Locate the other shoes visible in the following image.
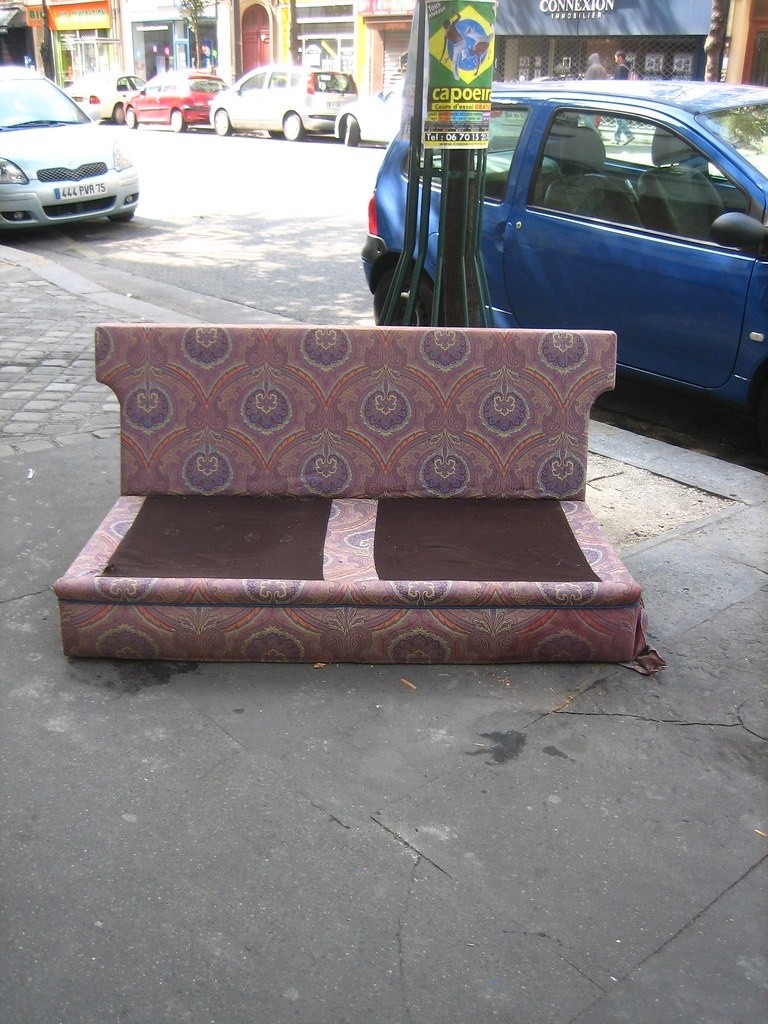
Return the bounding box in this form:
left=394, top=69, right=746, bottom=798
left=624, top=136, right=635, bottom=146
left=609, top=139, right=620, bottom=145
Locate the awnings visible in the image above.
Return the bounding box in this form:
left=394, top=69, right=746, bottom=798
left=0, top=6, right=27, bottom=27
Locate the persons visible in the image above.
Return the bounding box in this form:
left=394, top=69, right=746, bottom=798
left=194, top=44, right=215, bottom=68
left=610, top=51, right=635, bottom=146
left=148, top=43, right=170, bottom=78
left=584, top=53, right=607, bottom=131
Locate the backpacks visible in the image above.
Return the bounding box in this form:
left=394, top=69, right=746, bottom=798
left=620, top=63, right=643, bottom=81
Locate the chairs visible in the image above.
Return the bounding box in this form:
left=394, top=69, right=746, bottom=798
left=638, top=123, right=725, bottom=242
left=544, top=125, right=645, bottom=229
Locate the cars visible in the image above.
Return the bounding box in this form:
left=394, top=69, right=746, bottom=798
left=360, top=78, right=767, bottom=456
left=64, top=72, right=147, bottom=125
left=1, top=64, right=140, bottom=243
left=209, top=63, right=359, bottom=141
left=333, top=82, right=530, bottom=148
left=123, top=69, right=227, bottom=133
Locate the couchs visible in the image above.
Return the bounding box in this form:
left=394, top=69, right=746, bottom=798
left=52, top=322, right=666, bottom=680
